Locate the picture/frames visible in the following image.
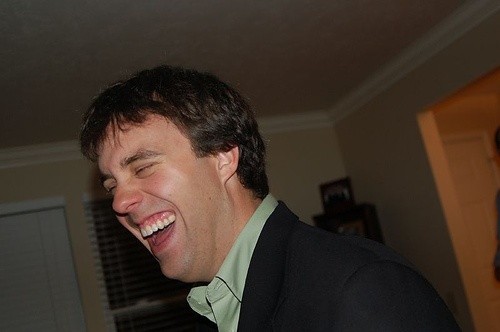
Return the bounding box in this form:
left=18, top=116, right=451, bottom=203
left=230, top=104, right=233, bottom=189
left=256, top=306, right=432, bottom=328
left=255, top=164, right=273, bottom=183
left=320, top=177, right=354, bottom=215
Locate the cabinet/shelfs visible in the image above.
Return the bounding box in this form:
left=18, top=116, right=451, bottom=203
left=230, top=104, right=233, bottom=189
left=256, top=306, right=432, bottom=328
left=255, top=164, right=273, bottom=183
left=312, top=203, right=384, bottom=243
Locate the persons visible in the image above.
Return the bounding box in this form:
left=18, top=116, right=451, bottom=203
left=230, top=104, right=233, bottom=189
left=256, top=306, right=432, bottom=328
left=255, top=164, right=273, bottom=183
left=80, top=62, right=462, bottom=331
